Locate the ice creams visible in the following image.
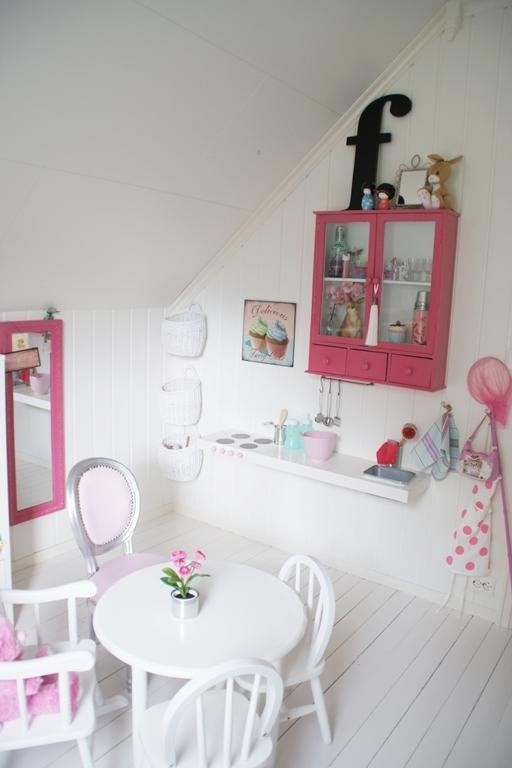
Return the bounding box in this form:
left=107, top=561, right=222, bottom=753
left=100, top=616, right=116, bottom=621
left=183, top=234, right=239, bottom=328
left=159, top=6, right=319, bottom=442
left=265, top=319, right=289, bottom=359
left=248, top=316, right=267, bottom=350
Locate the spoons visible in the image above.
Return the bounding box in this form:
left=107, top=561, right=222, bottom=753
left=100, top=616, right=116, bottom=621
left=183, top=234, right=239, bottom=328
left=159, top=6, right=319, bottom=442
left=314, top=392, right=343, bottom=427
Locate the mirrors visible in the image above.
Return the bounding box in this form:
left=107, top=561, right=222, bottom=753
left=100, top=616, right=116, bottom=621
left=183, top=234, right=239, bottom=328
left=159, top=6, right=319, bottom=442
left=392, top=154, right=433, bottom=208
left=0, top=319, right=66, bottom=526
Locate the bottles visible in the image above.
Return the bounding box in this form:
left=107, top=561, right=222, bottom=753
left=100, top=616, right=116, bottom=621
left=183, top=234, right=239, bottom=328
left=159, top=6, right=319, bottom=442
left=329, top=225, right=345, bottom=277
left=285, top=412, right=313, bottom=451
left=341, top=251, right=351, bottom=277
left=411, top=290, right=428, bottom=344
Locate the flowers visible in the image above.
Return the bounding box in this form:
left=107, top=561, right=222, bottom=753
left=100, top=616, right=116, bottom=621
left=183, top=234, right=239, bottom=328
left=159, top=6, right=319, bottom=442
left=159, top=550, right=211, bottom=598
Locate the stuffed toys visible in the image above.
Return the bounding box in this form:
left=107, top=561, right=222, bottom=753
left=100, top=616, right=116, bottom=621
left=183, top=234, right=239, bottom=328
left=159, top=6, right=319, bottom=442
left=336, top=294, right=365, bottom=339
left=375, top=183, right=396, bottom=210
left=418, top=154, right=464, bottom=209
left=361, top=182, right=374, bottom=210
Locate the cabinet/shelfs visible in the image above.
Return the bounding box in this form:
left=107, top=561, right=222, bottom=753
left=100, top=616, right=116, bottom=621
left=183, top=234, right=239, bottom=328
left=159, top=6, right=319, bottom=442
left=304, top=209, right=461, bottom=391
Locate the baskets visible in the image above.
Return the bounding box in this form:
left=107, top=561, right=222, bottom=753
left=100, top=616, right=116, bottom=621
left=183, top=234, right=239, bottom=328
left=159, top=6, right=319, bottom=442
left=160, top=366, right=201, bottom=427
left=162, top=303, right=206, bottom=357
left=158, top=425, right=203, bottom=482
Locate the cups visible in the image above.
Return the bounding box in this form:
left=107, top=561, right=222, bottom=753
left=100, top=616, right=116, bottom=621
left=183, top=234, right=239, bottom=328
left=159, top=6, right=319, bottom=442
left=301, top=430, right=337, bottom=462
left=355, top=255, right=367, bottom=278
left=28, top=374, right=49, bottom=393
left=266, top=337, right=288, bottom=358
left=406, top=255, right=432, bottom=282
left=274, top=425, right=286, bottom=446
left=248, top=331, right=266, bottom=350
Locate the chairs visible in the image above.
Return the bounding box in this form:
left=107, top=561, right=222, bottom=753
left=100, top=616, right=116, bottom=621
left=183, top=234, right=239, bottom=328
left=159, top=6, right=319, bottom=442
left=136, top=660, right=284, bottom=768
left=65, top=457, right=173, bottom=692
left=233, top=554, right=337, bottom=744
left=0, top=580, right=98, bottom=768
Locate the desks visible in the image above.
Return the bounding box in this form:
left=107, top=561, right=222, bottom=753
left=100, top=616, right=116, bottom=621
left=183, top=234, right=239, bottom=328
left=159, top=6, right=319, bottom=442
left=92, top=560, right=308, bottom=768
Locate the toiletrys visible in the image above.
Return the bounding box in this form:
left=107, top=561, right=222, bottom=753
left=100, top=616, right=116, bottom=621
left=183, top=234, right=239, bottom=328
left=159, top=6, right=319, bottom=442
left=410, top=291, right=430, bottom=346
left=299, top=413, right=312, bottom=449
left=284, top=418, right=299, bottom=449
left=384, top=256, right=432, bottom=283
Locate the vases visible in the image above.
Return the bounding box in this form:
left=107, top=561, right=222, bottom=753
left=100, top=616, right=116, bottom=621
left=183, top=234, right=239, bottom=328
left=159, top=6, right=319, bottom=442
left=170, top=587, right=200, bottom=620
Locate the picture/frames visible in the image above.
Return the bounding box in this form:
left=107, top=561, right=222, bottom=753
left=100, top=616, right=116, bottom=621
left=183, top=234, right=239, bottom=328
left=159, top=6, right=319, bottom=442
left=241, top=299, right=297, bottom=367
